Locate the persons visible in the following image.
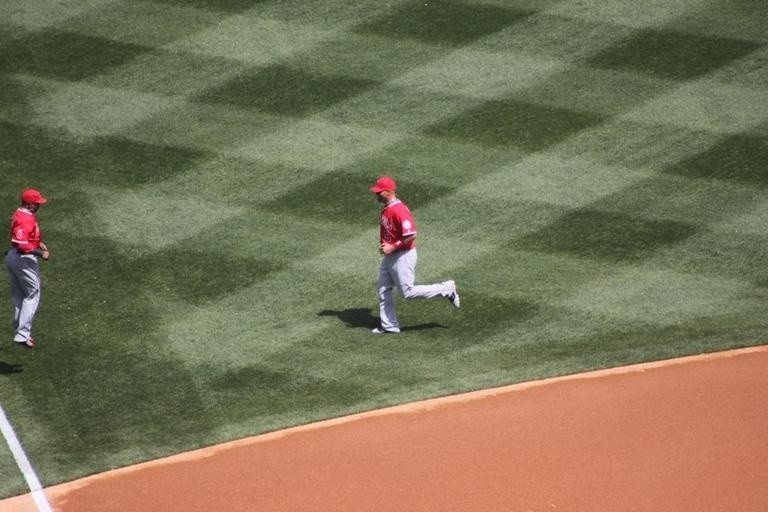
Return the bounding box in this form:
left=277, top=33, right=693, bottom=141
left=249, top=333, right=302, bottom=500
left=5, top=189, right=50, bottom=349
left=369, top=177, right=461, bottom=334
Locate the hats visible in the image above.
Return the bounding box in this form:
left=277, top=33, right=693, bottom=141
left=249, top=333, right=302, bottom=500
left=367, top=175, right=398, bottom=194
left=22, top=189, right=48, bottom=206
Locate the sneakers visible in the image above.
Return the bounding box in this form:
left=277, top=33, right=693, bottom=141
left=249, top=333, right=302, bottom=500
left=372, top=325, right=400, bottom=333
left=13, top=335, right=35, bottom=350
left=445, top=279, right=460, bottom=309
